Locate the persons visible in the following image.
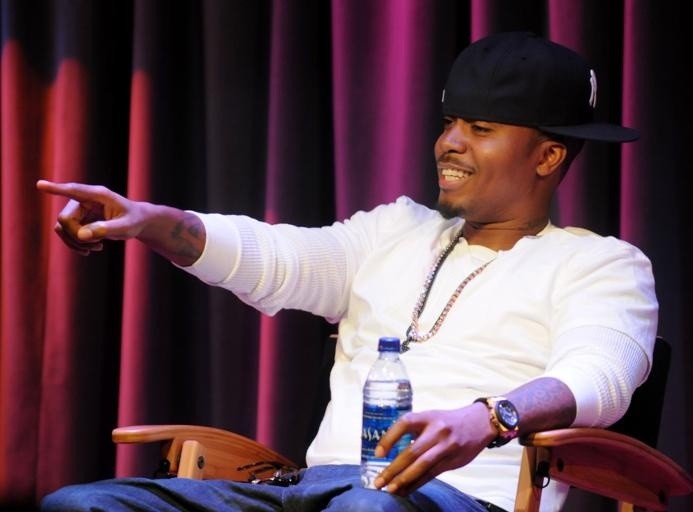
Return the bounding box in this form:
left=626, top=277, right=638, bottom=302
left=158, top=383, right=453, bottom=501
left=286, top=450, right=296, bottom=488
left=35, top=31, right=659, bottom=512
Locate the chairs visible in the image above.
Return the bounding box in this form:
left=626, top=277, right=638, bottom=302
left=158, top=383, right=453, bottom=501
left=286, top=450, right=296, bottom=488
left=109, top=337, right=691, bottom=512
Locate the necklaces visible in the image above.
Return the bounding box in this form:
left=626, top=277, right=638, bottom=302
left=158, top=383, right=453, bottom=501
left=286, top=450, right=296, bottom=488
left=401, top=220, right=499, bottom=353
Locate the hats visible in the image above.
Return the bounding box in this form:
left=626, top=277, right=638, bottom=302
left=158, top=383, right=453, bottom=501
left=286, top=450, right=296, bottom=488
left=442, top=35, right=641, bottom=142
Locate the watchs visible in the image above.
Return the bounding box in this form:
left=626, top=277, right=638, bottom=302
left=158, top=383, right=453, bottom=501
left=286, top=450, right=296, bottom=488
left=473, top=395, right=520, bottom=449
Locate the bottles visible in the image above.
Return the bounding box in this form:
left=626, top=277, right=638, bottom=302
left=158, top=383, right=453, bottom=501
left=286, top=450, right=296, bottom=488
left=359, top=338, right=414, bottom=491
